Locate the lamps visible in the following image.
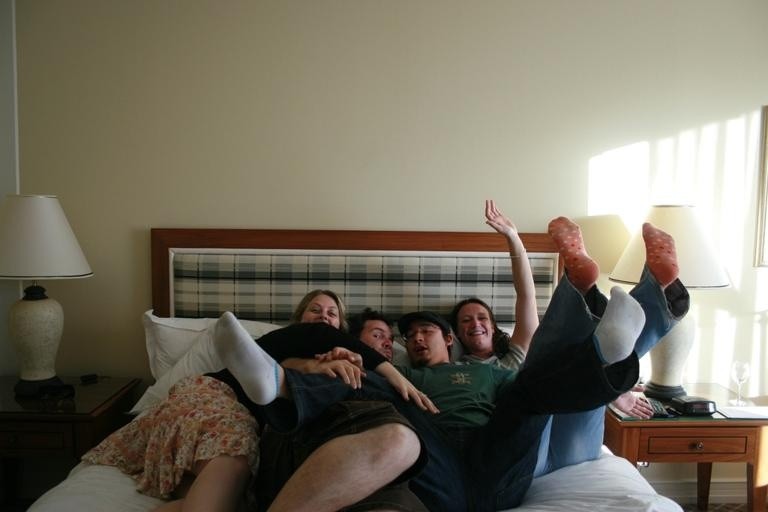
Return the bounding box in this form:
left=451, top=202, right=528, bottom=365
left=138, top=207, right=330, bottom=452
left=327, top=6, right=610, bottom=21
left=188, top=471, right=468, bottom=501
left=609, top=204, right=728, bottom=400
left=0, top=195, right=93, bottom=408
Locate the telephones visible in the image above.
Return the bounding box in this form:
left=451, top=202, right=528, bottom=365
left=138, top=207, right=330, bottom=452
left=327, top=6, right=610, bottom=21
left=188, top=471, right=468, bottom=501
left=644, top=397, right=669, bottom=418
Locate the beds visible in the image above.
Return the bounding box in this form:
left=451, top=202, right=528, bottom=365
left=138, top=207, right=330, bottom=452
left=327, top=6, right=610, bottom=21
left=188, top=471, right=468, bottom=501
left=25, top=228, right=683, bottom=512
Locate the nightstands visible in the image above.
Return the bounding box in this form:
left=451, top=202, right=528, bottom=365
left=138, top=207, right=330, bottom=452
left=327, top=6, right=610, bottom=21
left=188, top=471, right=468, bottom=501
left=0, top=377, right=141, bottom=512
left=606, top=385, right=767, bottom=512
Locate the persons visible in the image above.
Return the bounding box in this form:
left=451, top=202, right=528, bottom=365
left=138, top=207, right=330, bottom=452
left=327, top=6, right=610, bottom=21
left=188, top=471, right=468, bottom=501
left=73, top=198, right=689, bottom=512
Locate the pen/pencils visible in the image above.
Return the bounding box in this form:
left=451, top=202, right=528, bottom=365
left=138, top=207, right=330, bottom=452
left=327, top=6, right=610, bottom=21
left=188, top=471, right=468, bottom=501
left=666, top=407, right=682, bottom=416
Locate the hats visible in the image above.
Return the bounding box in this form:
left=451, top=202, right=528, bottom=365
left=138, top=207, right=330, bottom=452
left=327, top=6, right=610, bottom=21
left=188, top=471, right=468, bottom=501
left=397, top=310, right=451, bottom=338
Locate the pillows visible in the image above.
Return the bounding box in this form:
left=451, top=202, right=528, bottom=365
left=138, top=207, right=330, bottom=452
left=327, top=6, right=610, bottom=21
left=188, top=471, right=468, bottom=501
left=142, top=309, right=283, bottom=379
left=127, top=325, right=230, bottom=415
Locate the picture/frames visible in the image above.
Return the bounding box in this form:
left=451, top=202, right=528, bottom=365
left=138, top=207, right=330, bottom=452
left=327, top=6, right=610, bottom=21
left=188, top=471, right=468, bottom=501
left=754, top=106, right=768, bottom=268
